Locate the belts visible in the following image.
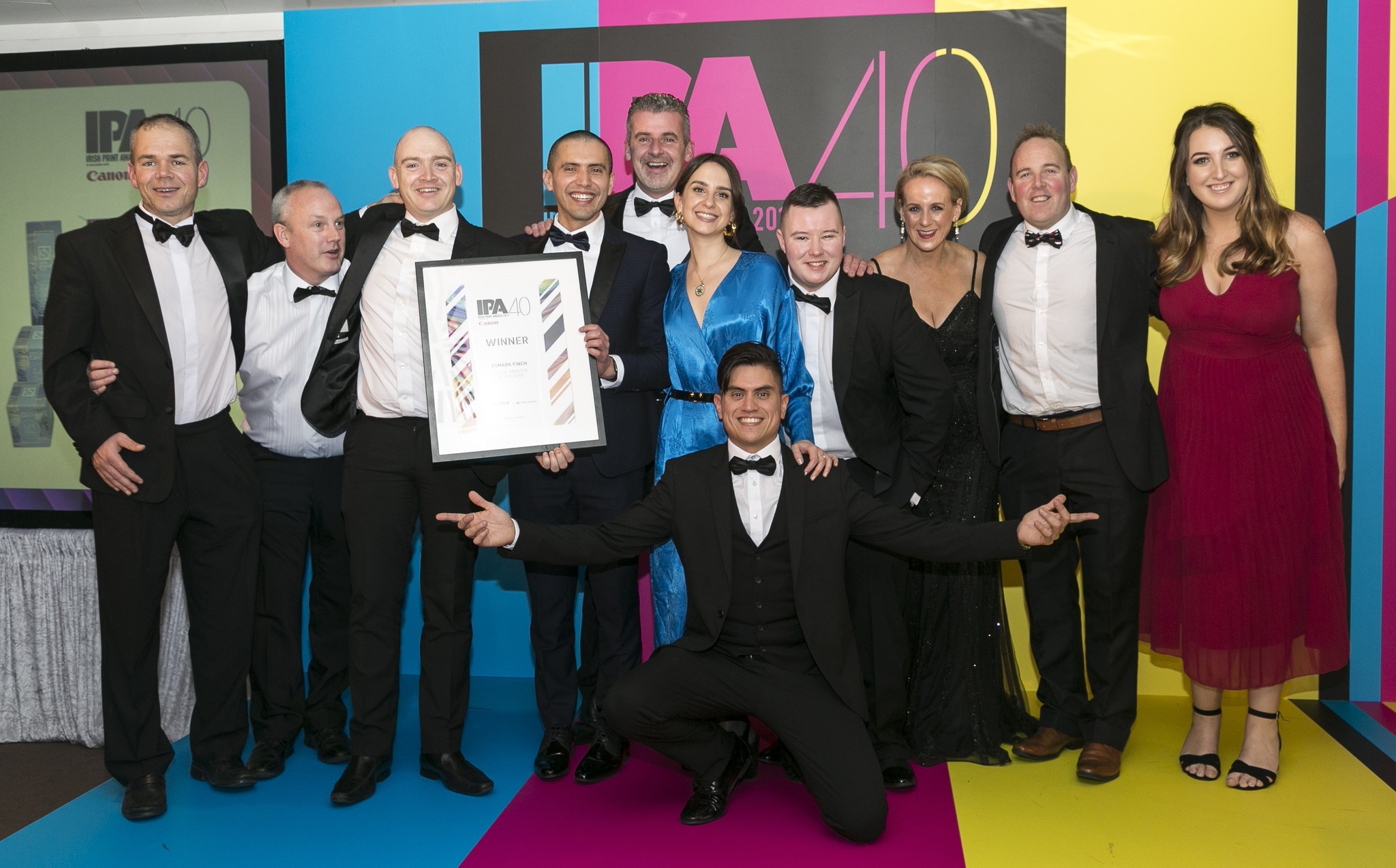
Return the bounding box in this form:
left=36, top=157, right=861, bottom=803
left=670, top=388, right=720, bottom=403
left=1010, top=409, right=1101, bottom=431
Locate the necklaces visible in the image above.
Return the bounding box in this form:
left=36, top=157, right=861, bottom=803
left=690, top=244, right=728, bottom=296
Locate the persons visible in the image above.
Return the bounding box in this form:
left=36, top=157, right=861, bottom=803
left=976, top=125, right=1167, bottom=781
left=1153, top=103, right=1349, bottom=789
left=42, top=115, right=404, bottom=818
left=88, top=180, right=353, bottom=779
left=649, top=154, right=839, bottom=652
left=758, top=183, right=947, bottom=790
left=302, top=126, right=507, bottom=805
left=525, top=93, right=875, bottom=744
left=865, top=155, right=1038, bottom=768
left=507, top=130, right=672, bottom=782
left=433, top=339, right=1100, bottom=845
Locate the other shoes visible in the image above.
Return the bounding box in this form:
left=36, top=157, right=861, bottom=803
left=576, top=719, right=596, bottom=746
left=879, top=757, right=917, bottom=793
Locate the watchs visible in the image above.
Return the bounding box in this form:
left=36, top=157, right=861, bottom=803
left=606, top=359, right=618, bottom=381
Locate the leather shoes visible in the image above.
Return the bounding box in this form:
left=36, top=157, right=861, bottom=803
left=1012, top=727, right=1078, bottom=760
left=122, top=772, right=167, bottom=821
left=760, top=740, right=787, bottom=766
left=190, top=751, right=256, bottom=789
left=246, top=738, right=295, bottom=780
left=330, top=754, right=392, bottom=805
left=304, top=729, right=352, bottom=763
left=681, top=746, right=753, bottom=823
left=575, top=727, right=631, bottom=783
left=534, top=727, right=572, bottom=781
left=420, top=749, right=494, bottom=795
left=1075, top=743, right=1121, bottom=781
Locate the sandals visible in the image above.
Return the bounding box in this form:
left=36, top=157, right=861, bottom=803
left=1179, top=704, right=1222, bottom=782
left=1227, top=707, right=1286, bottom=791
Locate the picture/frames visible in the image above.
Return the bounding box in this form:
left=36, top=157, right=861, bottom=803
left=0, top=37, right=295, bottom=529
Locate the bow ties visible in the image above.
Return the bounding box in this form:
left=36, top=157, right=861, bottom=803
left=790, top=283, right=831, bottom=315
left=136, top=205, right=196, bottom=248
left=401, top=218, right=439, bottom=241
left=1024, top=230, right=1062, bottom=249
left=549, top=224, right=590, bottom=251
left=634, top=197, right=677, bottom=217
left=294, top=286, right=335, bottom=303
left=729, top=454, right=777, bottom=476
